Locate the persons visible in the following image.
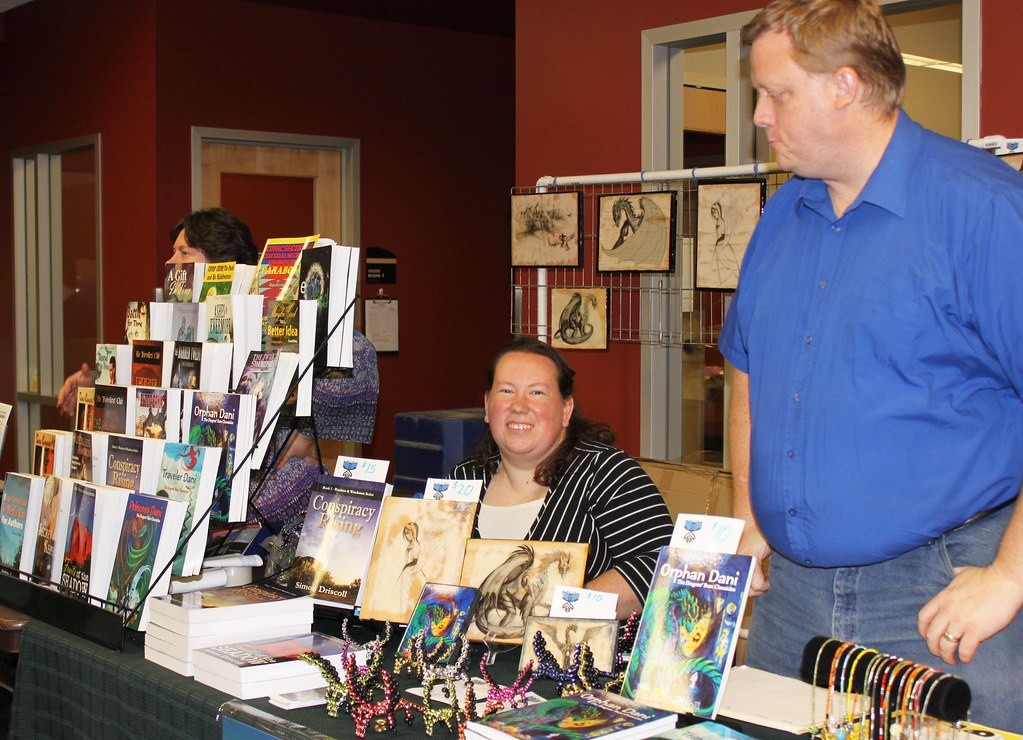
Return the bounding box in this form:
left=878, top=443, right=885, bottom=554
left=718, top=1, right=1023, bottom=731
left=452, top=340, right=676, bottom=618
left=55, top=209, right=261, bottom=424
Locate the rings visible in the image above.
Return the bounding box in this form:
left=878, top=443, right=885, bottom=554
left=944, top=632, right=961, bottom=643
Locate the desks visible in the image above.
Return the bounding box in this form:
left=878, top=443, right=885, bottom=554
left=0, top=606, right=821, bottom=740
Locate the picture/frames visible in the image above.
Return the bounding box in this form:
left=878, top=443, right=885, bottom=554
left=597, top=190, right=677, bottom=272
left=695, top=178, right=766, bottom=293
left=509, top=191, right=584, bottom=268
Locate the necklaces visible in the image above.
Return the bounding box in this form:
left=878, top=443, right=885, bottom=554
left=810, top=636, right=972, bottom=740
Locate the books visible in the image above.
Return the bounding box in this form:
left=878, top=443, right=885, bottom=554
left=0, top=236, right=362, bottom=649
left=285, top=475, right=395, bottom=612
left=621, top=546, right=757, bottom=721
